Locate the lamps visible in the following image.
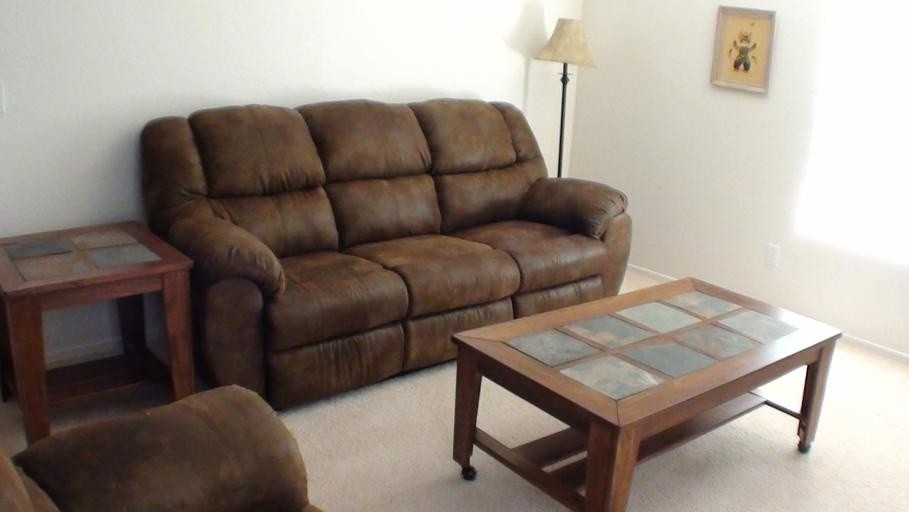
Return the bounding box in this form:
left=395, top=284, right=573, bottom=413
left=534, top=17, right=598, bottom=179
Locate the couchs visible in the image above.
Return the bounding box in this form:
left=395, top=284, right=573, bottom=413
left=138, top=98, right=632, bottom=412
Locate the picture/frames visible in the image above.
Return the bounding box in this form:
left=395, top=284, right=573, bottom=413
left=711, top=5, right=776, bottom=95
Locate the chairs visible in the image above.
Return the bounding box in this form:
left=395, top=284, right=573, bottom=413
left=1, top=385, right=324, bottom=512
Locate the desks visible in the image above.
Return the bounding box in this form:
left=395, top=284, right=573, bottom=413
left=1, top=219, right=196, bottom=445
